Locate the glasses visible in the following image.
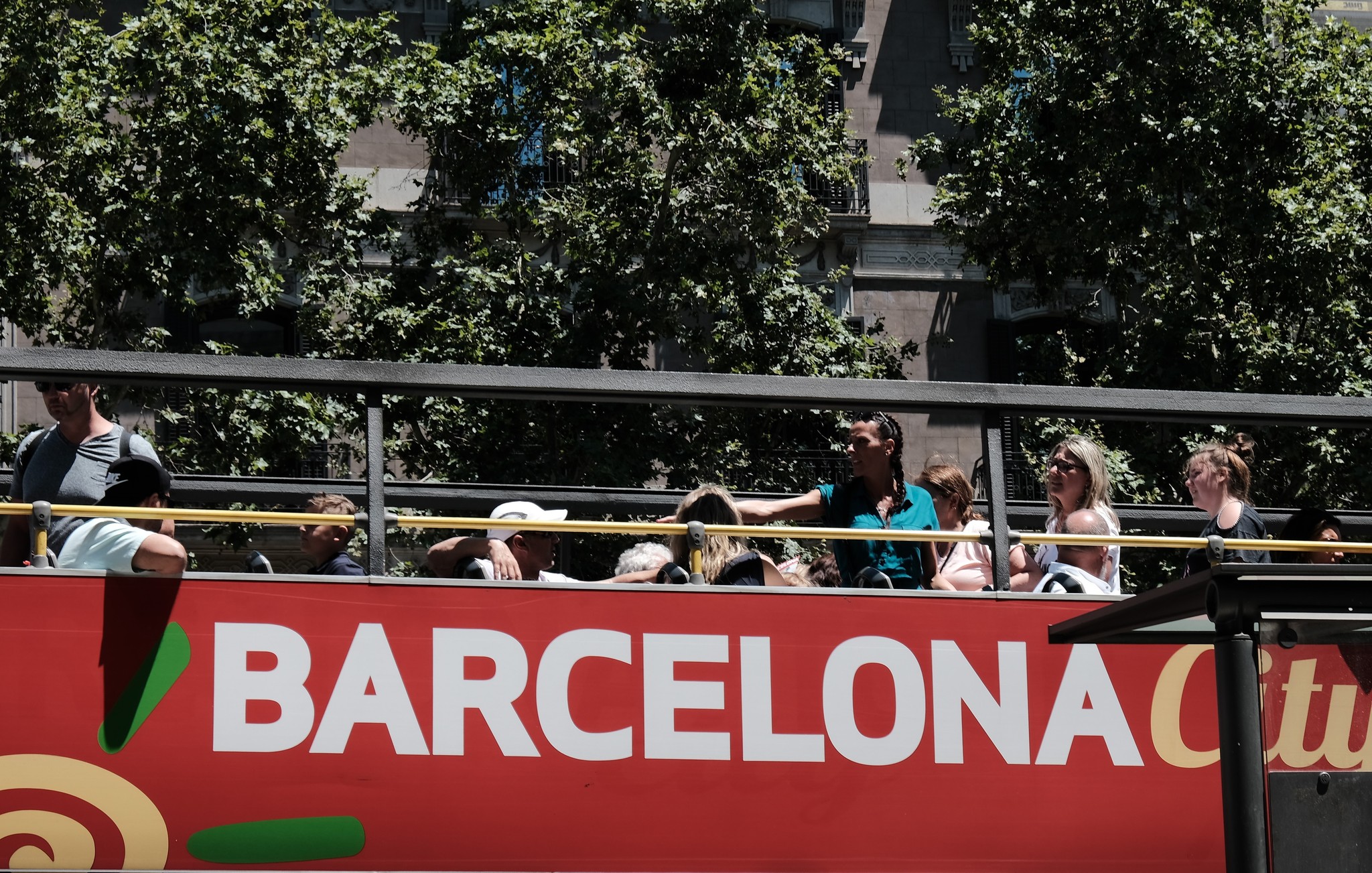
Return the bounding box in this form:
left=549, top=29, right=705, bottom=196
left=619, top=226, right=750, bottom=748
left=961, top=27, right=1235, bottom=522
left=1046, top=459, right=1083, bottom=473
left=34, top=381, right=75, bottom=392
left=1328, top=538, right=1340, bottom=542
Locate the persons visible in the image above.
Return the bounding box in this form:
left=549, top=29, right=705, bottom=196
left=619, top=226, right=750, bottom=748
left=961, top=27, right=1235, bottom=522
left=0, top=376, right=190, bottom=575
left=298, top=493, right=371, bottom=577
left=414, top=409, right=1347, bottom=595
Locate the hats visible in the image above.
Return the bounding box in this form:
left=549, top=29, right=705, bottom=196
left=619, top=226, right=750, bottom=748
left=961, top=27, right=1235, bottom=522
left=487, top=501, right=568, bottom=541
left=92, top=456, right=173, bottom=506
